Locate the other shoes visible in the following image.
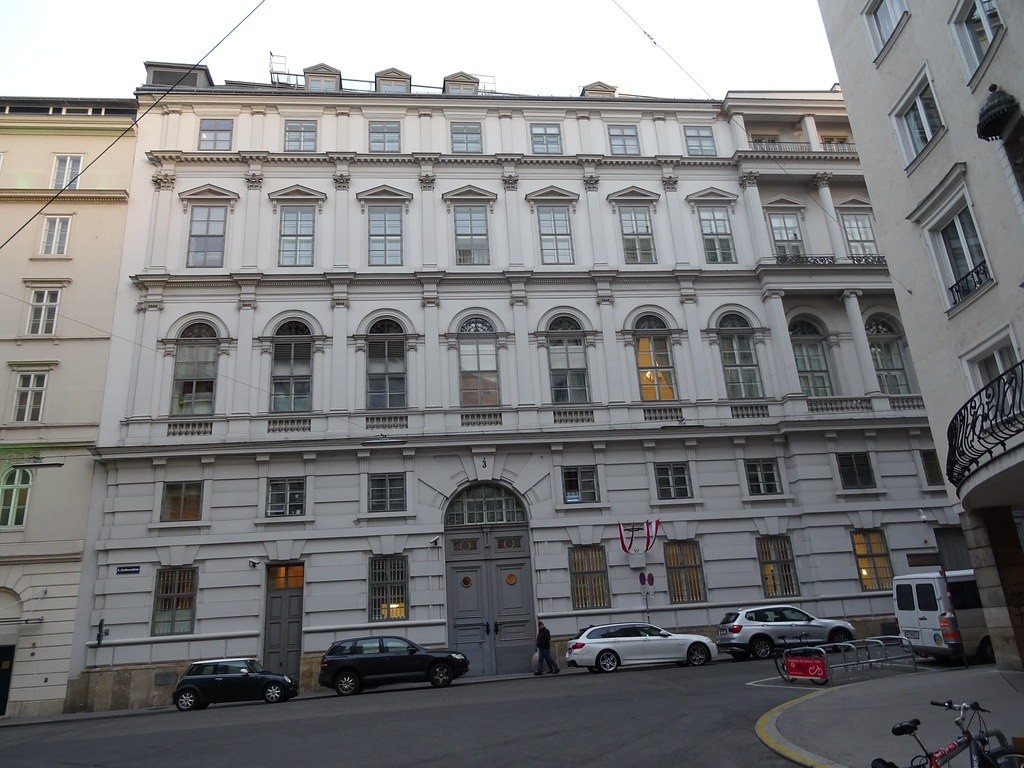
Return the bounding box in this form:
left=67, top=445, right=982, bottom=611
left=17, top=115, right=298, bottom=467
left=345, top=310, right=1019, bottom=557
left=553, top=669, right=560, bottom=674
left=546, top=671, right=554, bottom=674
left=534, top=672, right=543, bottom=675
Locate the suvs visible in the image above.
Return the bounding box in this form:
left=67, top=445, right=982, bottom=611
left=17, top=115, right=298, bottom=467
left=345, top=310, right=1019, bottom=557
left=172, top=656, right=300, bottom=712
left=318, top=634, right=470, bottom=696
left=715, top=603, right=857, bottom=661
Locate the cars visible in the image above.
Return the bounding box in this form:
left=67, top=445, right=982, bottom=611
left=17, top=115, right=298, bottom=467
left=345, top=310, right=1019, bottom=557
left=565, top=620, right=718, bottom=675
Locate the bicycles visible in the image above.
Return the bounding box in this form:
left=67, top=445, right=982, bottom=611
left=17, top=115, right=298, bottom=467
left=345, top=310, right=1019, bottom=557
left=871, top=698, right=1024, bottom=768
left=772, top=633, right=833, bottom=686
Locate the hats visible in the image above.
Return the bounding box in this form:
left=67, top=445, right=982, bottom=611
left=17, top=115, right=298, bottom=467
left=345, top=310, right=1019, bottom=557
left=538, top=622, right=543, bottom=626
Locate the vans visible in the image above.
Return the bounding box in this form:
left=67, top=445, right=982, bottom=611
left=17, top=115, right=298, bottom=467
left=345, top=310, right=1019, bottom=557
left=892, top=568, right=996, bottom=666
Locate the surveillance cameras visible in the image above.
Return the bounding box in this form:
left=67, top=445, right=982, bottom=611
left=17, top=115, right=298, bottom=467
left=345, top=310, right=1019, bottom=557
left=429, top=536, right=439, bottom=544
left=921, top=515, right=927, bottom=523
left=249, top=558, right=260, bottom=564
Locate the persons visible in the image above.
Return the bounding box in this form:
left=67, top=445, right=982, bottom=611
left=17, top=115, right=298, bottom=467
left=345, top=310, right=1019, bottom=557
left=534, top=620, right=561, bottom=675
